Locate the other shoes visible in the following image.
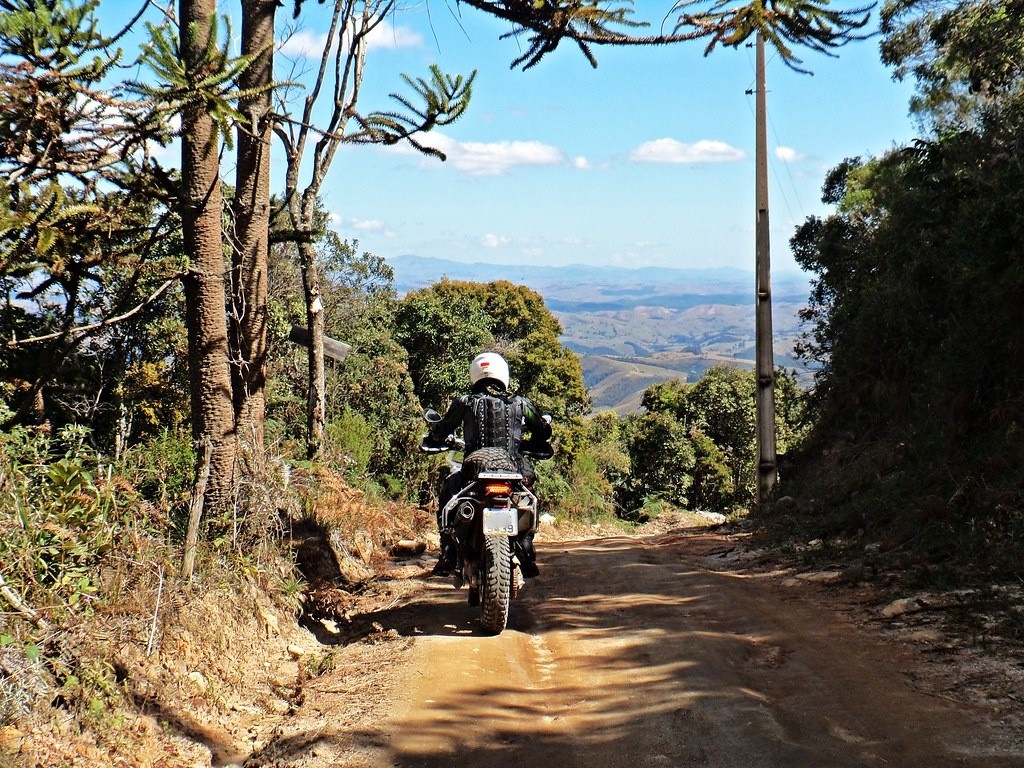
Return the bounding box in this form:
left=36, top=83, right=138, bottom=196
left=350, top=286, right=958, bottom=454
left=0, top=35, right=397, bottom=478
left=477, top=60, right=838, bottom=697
left=522, top=539, right=535, bottom=561
left=432, top=554, right=457, bottom=576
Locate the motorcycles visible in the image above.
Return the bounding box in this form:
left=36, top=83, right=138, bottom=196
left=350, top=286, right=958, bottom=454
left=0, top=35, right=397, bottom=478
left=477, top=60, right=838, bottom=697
left=417, top=408, right=552, bottom=637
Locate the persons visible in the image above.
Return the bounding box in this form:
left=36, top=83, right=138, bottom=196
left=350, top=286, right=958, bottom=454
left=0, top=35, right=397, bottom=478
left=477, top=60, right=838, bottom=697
left=431, top=352, right=552, bottom=578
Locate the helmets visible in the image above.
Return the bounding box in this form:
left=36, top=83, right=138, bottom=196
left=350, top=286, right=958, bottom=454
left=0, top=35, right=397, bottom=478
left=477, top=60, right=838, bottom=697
left=469, top=352, right=510, bottom=391
